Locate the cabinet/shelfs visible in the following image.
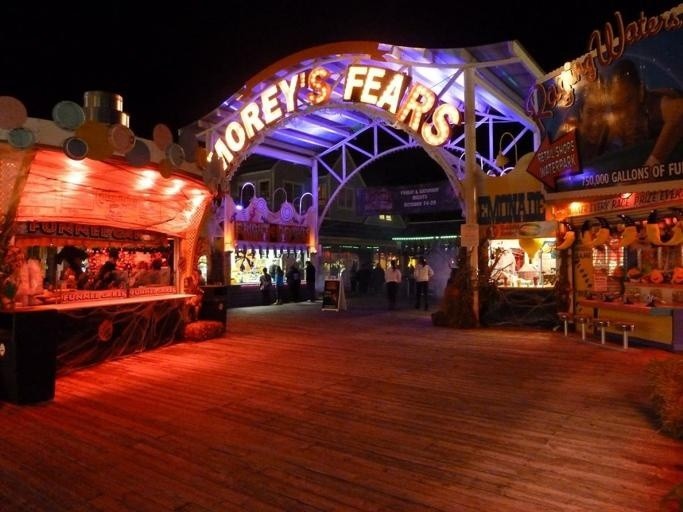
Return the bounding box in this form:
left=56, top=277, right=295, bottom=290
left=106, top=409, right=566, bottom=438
left=200, top=283, right=231, bottom=335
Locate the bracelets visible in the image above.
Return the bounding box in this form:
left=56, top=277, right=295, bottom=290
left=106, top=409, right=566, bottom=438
left=643, top=154, right=661, bottom=173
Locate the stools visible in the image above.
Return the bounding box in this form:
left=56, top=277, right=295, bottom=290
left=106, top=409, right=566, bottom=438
left=592, top=317, right=612, bottom=346
left=574, top=313, right=591, bottom=342
left=614, top=321, right=635, bottom=349
left=555, top=312, right=571, bottom=337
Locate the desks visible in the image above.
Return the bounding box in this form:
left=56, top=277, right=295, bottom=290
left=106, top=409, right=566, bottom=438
left=493, top=284, right=559, bottom=325
left=0, top=290, right=199, bottom=409
left=576, top=281, right=682, bottom=347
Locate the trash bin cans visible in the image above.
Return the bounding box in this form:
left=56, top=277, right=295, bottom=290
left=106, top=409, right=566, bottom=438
left=201, top=284, right=227, bottom=323
left=0, top=311, right=59, bottom=405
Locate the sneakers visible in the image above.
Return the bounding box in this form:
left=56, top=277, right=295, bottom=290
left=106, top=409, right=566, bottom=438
left=262, top=296, right=315, bottom=306
left=388, top=304, right=428, bottom=310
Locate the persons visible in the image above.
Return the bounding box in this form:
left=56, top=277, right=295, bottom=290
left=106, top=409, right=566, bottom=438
left=272, top=266, right=284, bottom=305
left=553, top=72, right=611, bottom=171
left=305, top=261, right=316, bottom=300
left=348, top=256, right=434, bottom=311
left=59, top=247, right=162, bottom=290
left=260, top=268, right=272, bottom=306
left=285, top=265, right=301, bottom=303
left=605, top=59, right=683, bottom=182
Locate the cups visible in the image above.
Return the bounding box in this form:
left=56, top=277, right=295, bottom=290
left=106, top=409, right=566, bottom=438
left=503, top=275, right=539, bottom=288
